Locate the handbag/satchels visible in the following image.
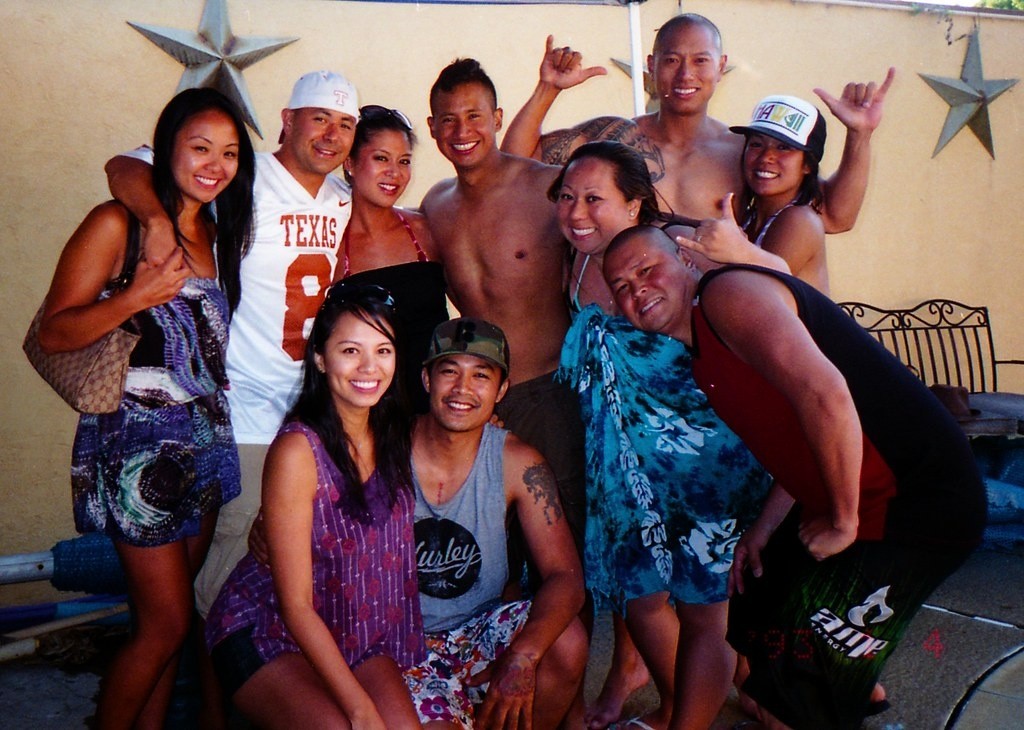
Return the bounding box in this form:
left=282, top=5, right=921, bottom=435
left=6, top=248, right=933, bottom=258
left=22, top=199, right=142, bottom=416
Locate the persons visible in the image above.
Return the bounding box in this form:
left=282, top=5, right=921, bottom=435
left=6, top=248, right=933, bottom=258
left=206, top=283, right=428, bottom=730
left=601, top=225, right=990, bottom=730
left=332, top=104, right=450, bottom=417
left=36, top=87, right=258, bottom=730
left=418, top=59, right=595, bottom=730
left=104, top=72, right=360, bottom=730
left=246, top=316, right=590, bottom=730
left=547, top=140, right=791, bottom=730
left=499, top=12, right=896, bottom=730
left=729, top=94, right=830, bottom=296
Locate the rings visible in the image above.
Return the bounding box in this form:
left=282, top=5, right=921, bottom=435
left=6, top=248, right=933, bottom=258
left=697, top=236, right=702, bottom=241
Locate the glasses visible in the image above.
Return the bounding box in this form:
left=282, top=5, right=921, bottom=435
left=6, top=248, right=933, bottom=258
left=358, top=105, right=413, bottom=131
left=327, top=285, right=395, bottom=312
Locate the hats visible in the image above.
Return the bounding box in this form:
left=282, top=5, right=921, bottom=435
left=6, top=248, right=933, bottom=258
left=277, top=71, right=361, bottom=145
left=422, top=318, right=511, bottom=377
left=729, top=95, right=827, bottom=163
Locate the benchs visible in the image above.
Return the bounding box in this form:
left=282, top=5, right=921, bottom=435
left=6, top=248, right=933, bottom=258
left=836, top=299, right=1024, bottom=437
